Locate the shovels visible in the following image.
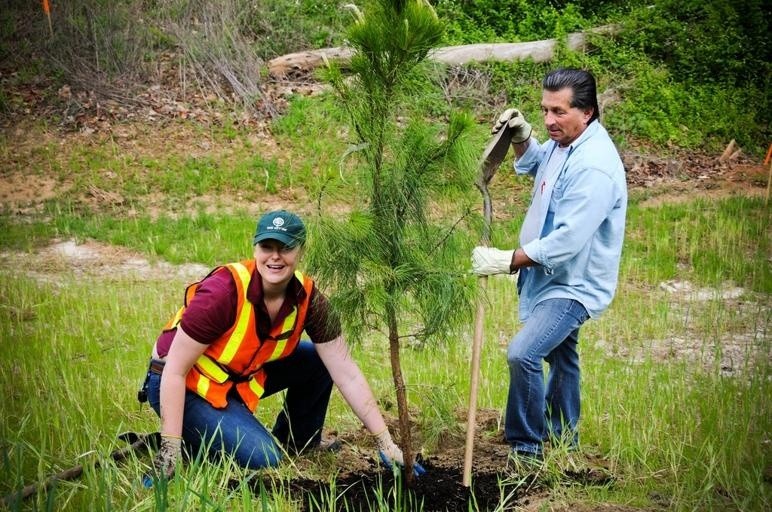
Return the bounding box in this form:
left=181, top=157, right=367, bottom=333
left=462, top=112, right=520, bottom=487
left=0, top=431, right=162, bottom=508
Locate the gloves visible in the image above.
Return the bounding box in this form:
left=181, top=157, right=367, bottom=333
left=369, top=425, right=426, bottom=478
left=143, top=433, right=184, bottom=488
left=470, top=245, right=520, bottom=277
left=491, top=108, right=533, bottom=144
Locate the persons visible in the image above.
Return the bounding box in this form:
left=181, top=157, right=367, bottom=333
left=142, top=207, right=427, bottom=493
left=468, top=66, right=629, bottom=459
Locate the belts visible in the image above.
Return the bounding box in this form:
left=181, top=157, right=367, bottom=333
left=149, top=362, right=163, bottom=375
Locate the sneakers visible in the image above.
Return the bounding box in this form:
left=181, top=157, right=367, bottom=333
left=318, top=438, right=342, bottom=454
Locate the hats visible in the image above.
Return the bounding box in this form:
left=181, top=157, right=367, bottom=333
left=252, top=208, right=307, bottom=249
